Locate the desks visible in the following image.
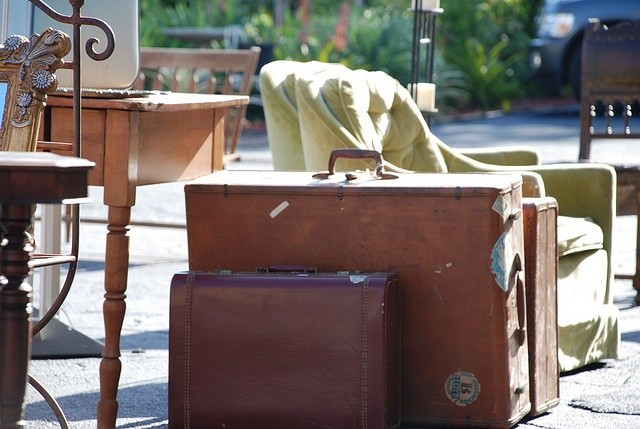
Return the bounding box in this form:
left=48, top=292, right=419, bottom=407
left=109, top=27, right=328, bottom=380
left=0, top=154, right=96, bottom=429
left=39, top=91, right=249, bottom=427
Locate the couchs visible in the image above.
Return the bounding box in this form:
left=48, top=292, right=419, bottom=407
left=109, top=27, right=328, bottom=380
left=259, top=59, right=343, bottom=173
left=295, top=69, right=621, bottom=374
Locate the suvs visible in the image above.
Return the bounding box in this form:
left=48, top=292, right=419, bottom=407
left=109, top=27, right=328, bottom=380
left=532, top=2, right=640, bottom=117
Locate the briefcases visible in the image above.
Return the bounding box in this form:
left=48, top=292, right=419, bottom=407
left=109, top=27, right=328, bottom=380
left=168, top=265, right=403, bottom=429
left=524, top=196, right=561, bottom=417
left=184, top=150, right=532, bottom=428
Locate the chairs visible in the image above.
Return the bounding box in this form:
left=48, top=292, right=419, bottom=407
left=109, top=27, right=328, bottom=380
left=132, top=45, right=260, bottom=171
left=580, top=23, right=639, bottom=306
left=0, top=0, right=116, bottom=428
